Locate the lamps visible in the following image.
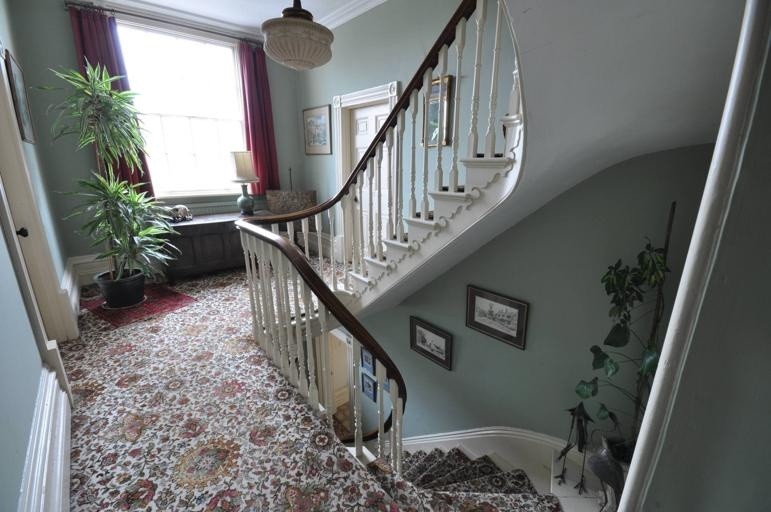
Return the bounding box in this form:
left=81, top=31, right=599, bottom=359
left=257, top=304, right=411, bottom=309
left=261, top=0, right=334, bottom=73
left=230, top=150, right=262, bottom=217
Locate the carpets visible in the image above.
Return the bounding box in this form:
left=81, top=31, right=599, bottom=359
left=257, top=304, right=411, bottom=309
left=79, top=280, right=201, bottom=327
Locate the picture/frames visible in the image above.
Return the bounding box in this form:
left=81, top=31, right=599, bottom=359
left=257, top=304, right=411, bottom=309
left=360, top=345, right=378, bottom=402
left=422, top=76, right=454, bottom=147
left=4, top=49, right=39, bottom=145
left=303, top=103, right=333, bottom=156
left=409, top=284, right=529, bottom=370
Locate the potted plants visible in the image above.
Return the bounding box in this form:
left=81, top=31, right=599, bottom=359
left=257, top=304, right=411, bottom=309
left=28, top=55, right=183, bottom=309
left=555, top=201, right=678, bottom=512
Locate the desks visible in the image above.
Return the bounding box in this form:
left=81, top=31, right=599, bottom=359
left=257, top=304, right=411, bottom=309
left=151, top=209, right=279, bottom=289
